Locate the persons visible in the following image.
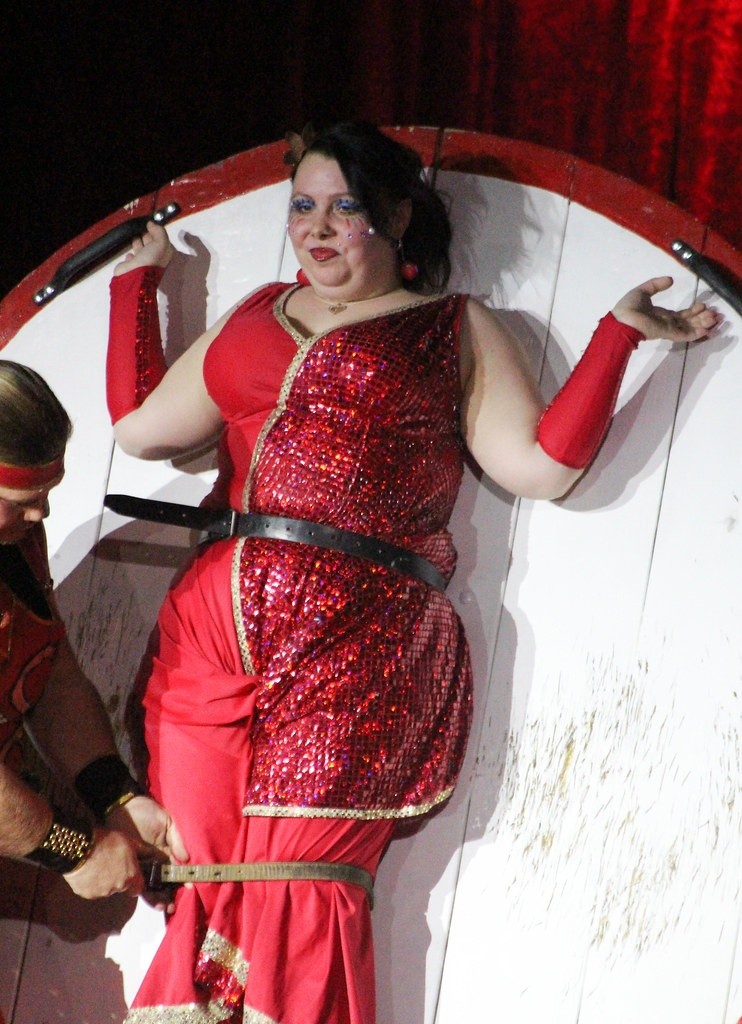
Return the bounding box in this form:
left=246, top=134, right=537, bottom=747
left=106, top=122, right=719, bottom=1024
left=0, top=359, right=189, bottom=914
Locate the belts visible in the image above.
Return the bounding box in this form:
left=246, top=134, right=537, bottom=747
left=138, top=860, right=375, bottom=911
left=103, top=494, right=446, bottom=594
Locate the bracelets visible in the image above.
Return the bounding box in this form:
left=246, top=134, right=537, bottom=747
left=22, top=804, right=96, bottom=874
left=73, top=753, right=144, bottom=821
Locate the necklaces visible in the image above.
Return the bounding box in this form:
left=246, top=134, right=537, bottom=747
left=313, top=287, right=406, bottom=315
